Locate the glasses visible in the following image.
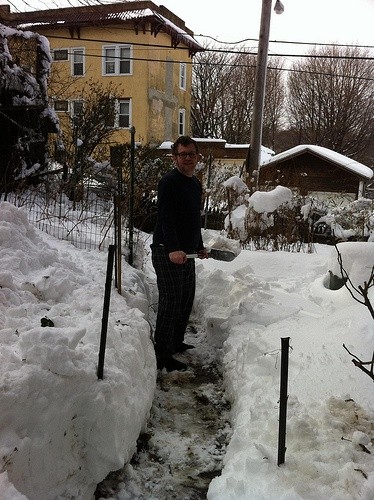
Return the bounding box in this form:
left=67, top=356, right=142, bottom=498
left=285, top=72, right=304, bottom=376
left=176, top=152, right=197, bottom=158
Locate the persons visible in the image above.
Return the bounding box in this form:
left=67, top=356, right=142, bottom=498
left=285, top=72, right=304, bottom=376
left=150, top=136, right=209, bottom=372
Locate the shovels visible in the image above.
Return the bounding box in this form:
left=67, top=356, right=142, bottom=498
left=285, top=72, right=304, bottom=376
left=186, top=249, right=237, bottom=262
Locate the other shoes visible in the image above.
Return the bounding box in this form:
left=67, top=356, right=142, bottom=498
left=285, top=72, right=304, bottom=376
left=157, top=357, right=188, bottom=373
left=171, top=342, right=195, bottom=355
